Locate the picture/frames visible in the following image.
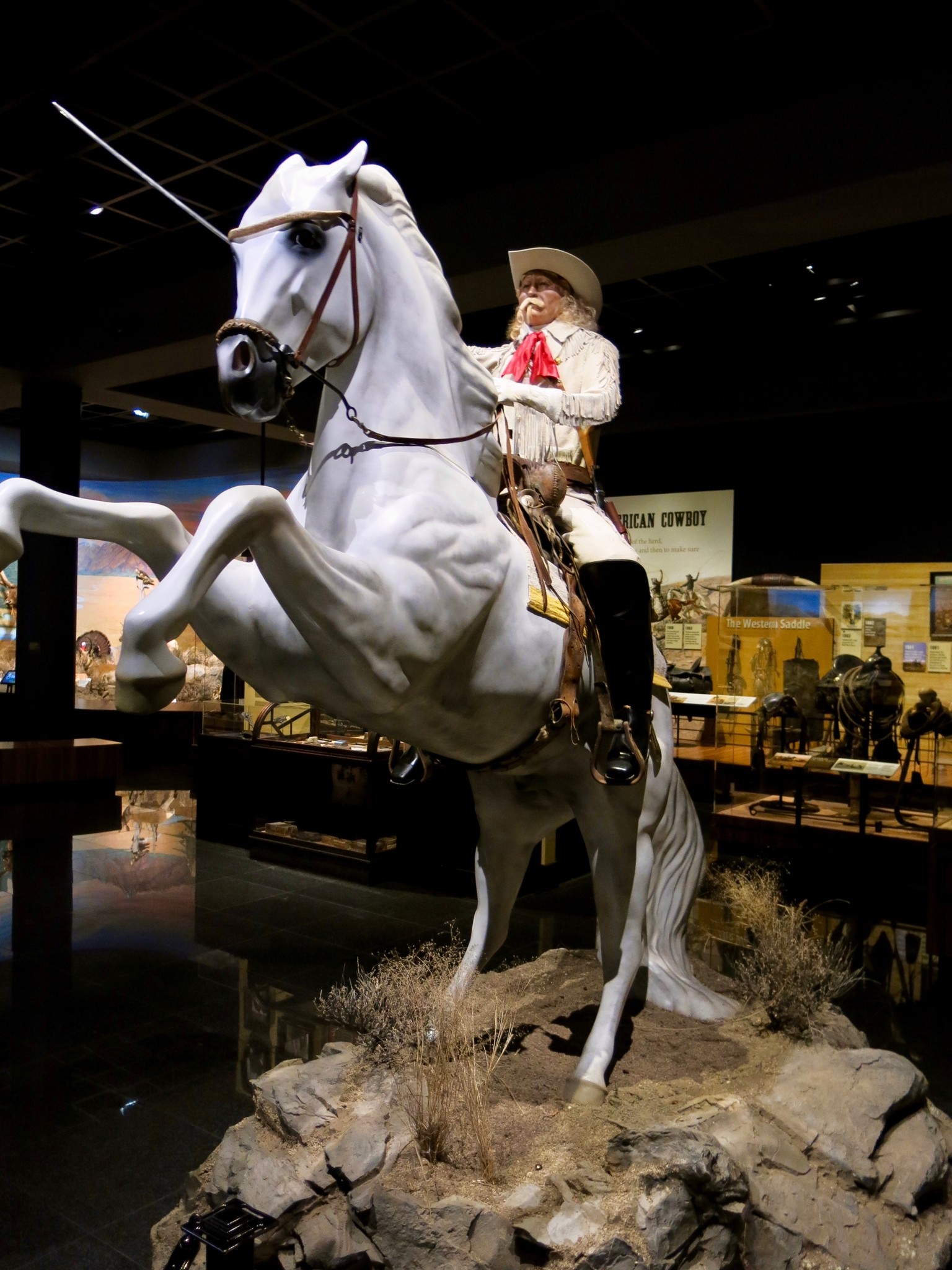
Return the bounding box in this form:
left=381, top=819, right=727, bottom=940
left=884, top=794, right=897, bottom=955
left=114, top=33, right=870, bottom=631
left=930, top=571, right=952, bottom=639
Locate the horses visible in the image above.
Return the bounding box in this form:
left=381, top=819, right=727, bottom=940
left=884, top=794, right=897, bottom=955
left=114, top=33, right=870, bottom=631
left=1, top=141, right=746, bottom=1105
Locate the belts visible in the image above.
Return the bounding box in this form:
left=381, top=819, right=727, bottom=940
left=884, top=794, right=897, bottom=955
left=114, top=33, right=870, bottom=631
left=553, top=460, right=593, bottom=492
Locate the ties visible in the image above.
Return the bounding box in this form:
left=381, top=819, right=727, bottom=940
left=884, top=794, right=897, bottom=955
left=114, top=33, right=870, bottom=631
left=501, top=331, right=560, bottom=382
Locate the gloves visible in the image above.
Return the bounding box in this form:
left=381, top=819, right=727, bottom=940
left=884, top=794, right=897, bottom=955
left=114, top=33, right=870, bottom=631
left=492, top=377, right=562, bottom=423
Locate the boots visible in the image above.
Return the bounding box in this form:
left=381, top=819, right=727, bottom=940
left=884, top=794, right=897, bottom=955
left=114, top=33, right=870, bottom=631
left=390, top=744, right=424, bottom=786
left=577, top=559, right=655, bottom=784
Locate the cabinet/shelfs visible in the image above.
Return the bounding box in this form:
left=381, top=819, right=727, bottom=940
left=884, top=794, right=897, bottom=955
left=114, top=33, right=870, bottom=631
left=246, top=700, right=408, bottom=877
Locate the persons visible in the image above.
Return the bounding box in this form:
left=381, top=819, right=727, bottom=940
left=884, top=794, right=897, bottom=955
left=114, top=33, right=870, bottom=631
left=650, top=569, right=701, bottom=620
left=848, top=608, right=856, bottom=624
left=0, top=570, right=16, bottom=589
left=466, top=248, right=655, bottom=784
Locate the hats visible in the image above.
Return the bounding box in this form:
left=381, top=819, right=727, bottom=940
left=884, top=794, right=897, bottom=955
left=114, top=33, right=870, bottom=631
left=508, top=247, right=603, bottom=323
